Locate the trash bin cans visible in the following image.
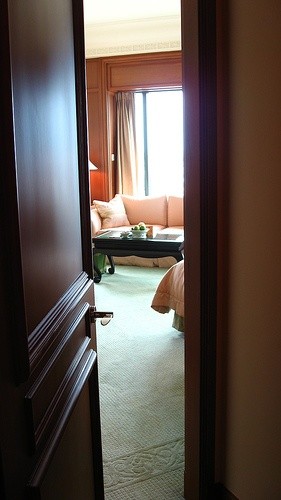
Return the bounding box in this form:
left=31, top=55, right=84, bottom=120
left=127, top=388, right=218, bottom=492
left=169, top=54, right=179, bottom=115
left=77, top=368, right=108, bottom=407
left=94, top=253, right=107, bottom=274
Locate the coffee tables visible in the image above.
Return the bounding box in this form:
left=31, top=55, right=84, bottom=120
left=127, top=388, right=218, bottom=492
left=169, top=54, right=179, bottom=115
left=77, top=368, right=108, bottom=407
left=92, top=228, right=184, bottom=274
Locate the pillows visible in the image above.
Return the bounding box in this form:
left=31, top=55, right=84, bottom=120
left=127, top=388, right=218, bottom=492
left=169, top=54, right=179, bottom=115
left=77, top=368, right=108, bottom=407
left=93, top=194, right=130, bottom=228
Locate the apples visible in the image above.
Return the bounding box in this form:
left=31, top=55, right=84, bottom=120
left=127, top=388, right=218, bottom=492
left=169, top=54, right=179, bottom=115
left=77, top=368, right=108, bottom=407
left=134, top=225, right=146, bottom=230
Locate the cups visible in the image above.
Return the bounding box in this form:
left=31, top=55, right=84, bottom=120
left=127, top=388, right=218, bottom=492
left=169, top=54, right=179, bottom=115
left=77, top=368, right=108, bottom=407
left=146, top=225, right=153, bottom=239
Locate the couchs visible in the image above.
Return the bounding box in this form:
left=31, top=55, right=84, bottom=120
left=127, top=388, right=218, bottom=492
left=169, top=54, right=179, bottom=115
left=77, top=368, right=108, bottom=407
left=91, top=194, right=184, bottom=268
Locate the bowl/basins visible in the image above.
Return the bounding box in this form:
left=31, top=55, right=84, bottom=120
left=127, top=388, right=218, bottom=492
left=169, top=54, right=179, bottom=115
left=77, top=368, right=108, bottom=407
left=131, top=228, right=149, bottom=239
